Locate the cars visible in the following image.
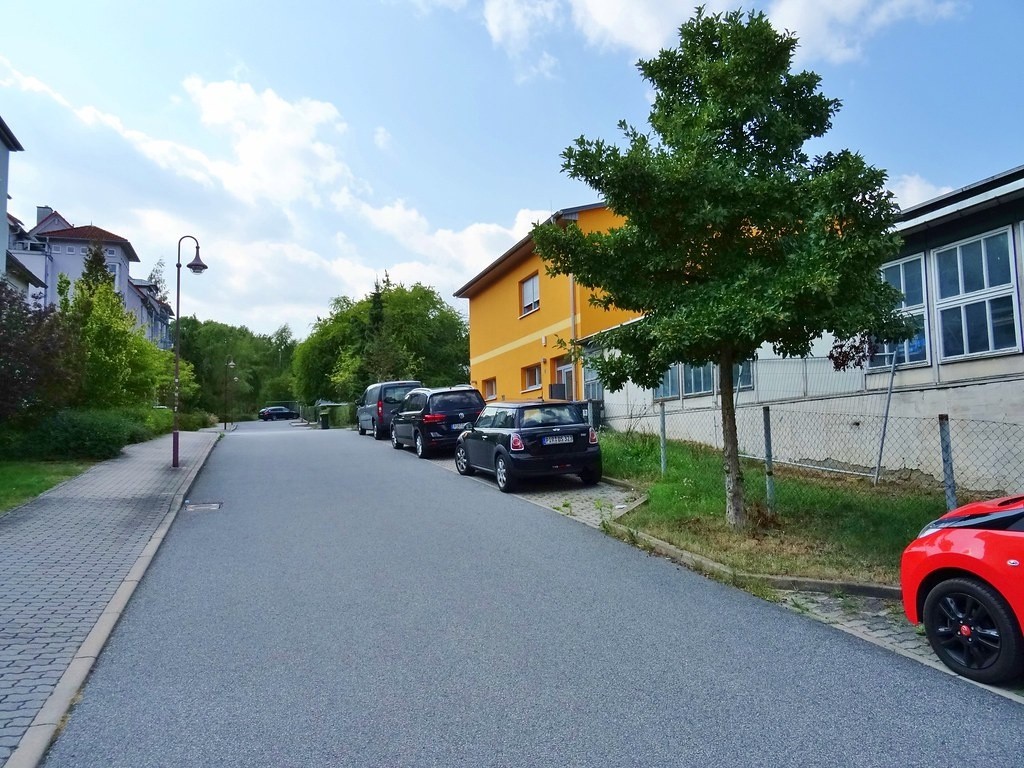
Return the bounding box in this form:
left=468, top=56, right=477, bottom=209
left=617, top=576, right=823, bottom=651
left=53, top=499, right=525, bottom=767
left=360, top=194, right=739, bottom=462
left=455, top=402, right=602, bottom=491
left=258, top=406, right=300, bottom=421
left=900, top=494, right=1024, bottom=685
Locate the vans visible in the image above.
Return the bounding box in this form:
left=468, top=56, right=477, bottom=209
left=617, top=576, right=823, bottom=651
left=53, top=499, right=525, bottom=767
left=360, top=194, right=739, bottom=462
left=355, top=381, right=422, bottom=440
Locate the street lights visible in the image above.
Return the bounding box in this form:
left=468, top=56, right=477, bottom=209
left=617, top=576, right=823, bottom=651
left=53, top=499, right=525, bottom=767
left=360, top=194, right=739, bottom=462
left=172, top=236, right=208, bottom=468
left=224, top=355, right=235, bottom=430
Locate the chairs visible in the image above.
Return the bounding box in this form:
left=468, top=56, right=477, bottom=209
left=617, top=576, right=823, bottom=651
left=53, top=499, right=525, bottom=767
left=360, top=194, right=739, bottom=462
left=541, top=410, right=556, bottom=424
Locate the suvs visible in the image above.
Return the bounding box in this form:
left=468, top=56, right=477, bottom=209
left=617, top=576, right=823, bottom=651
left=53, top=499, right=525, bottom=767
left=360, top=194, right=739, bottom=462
left=391, top=387, right=487, bottom=458
